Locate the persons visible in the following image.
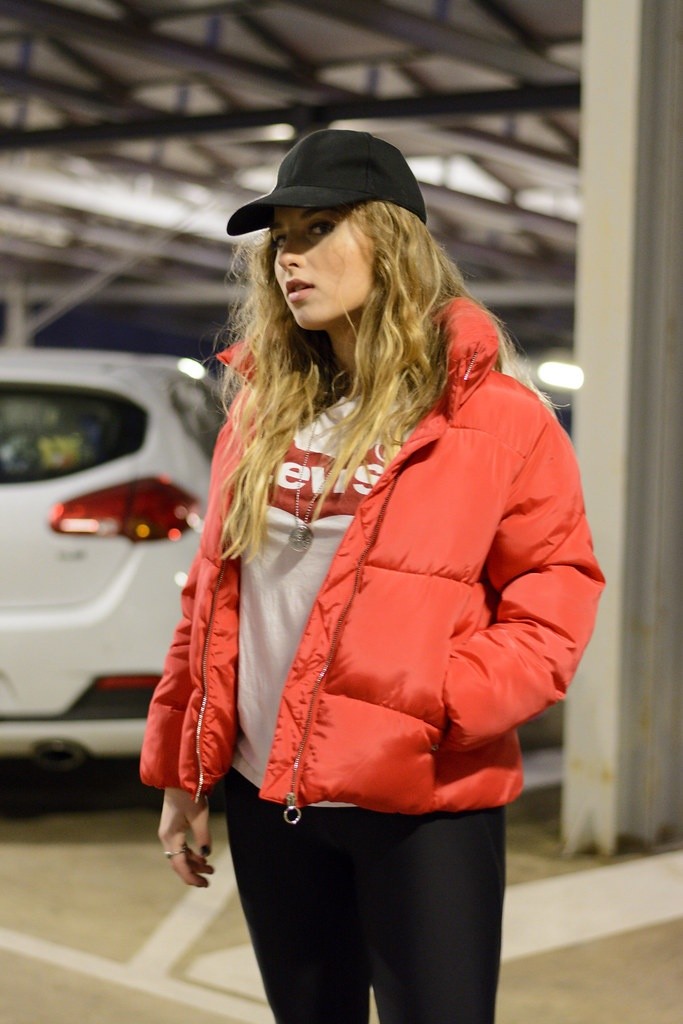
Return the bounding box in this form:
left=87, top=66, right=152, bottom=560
left=140, top=130, right=605, bottom=1024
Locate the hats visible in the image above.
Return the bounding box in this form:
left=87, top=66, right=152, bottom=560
left=227, top=129, right=428, bottom=236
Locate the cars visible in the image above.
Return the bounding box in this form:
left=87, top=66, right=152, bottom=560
left=0, top=344, right=228, bottom=811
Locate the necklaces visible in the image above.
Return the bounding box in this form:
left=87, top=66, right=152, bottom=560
left=289, top=403, right=332, bottom=553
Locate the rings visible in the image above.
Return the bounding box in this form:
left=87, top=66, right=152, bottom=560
left=166, top=850, right=186, bottom=858
